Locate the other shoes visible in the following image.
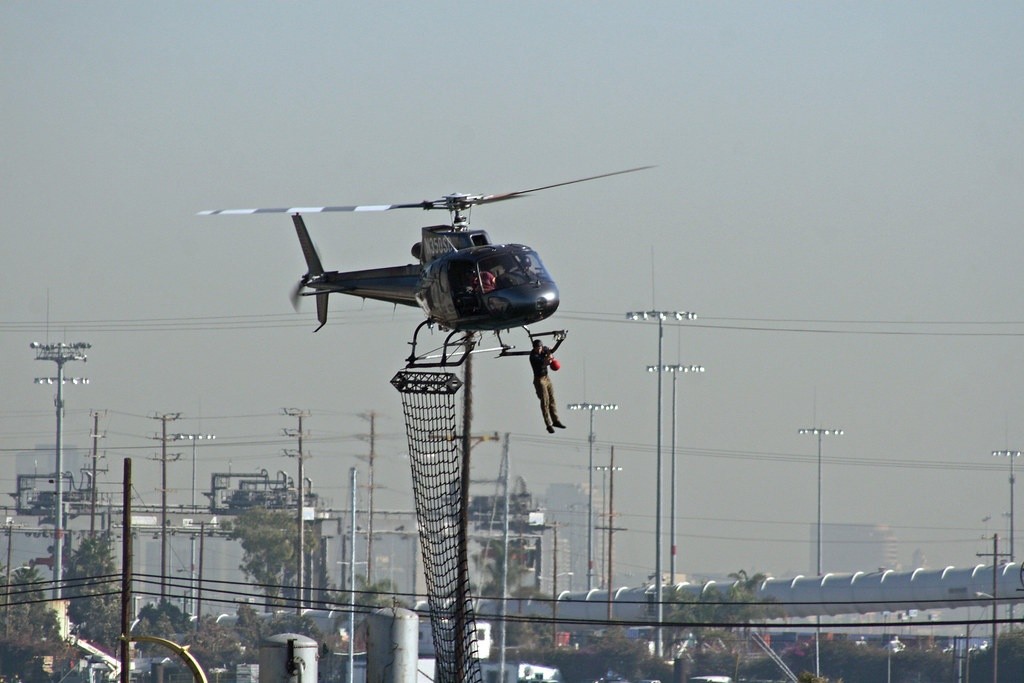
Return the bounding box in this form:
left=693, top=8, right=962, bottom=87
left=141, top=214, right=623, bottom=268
left=547, top=426, right=555, bottom=433
left=552, top=421, right=566, bottom=428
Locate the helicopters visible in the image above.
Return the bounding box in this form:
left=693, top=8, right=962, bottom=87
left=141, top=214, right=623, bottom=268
left=196, top=160, right=659, bottom=357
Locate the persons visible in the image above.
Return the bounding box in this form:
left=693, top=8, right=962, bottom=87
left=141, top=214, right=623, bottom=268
left=529, top=340, right=566, bottom=433
left=467, top=256, right=544, bottom=312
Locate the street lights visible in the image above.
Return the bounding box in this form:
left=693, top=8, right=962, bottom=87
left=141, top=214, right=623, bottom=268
left=569, top=401, right=621, bottom=592
left=176, top=433, right=217, bottom=517
left=589, top=463, right=625, bottom=587
left=992, top=449, right=1023, bottom=563
left=645, top=364, right=706, bottom=598
left=29, top=340, right=94, bottom=618
left=626, top=309, right=697, bottom=664
left=800, top=427, right=847, bottom=582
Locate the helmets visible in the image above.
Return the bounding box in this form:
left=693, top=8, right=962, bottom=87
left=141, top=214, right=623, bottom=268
left=533, top=339, right=543, bottom=347
